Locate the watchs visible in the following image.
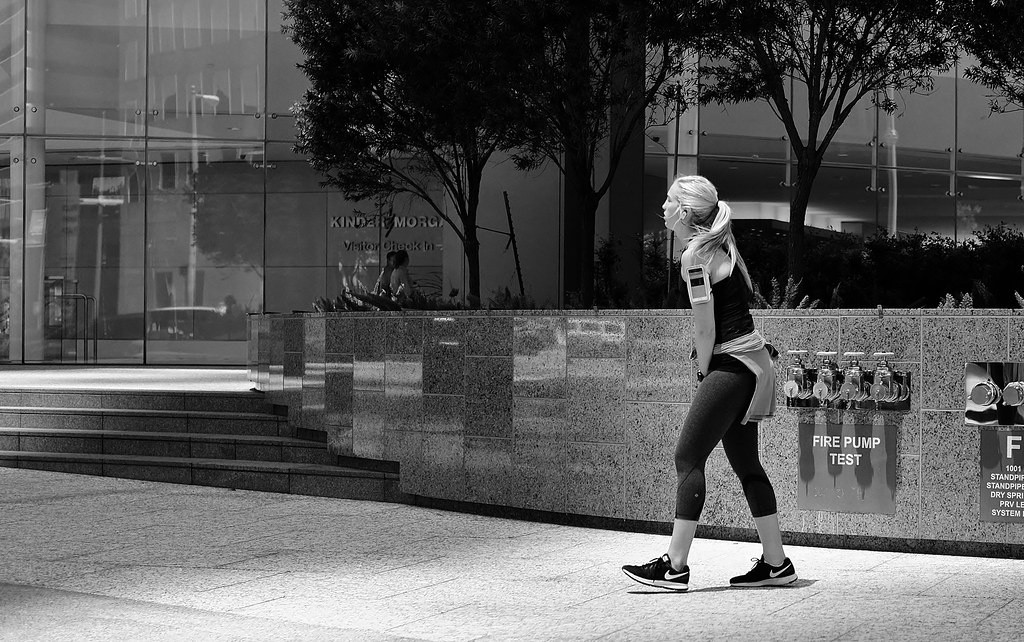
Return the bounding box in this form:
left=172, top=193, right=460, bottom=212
left=697, top=369, right=710, bottom=382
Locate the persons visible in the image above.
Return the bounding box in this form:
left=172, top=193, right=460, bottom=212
left=622, top=175, right=798, bottom=590
left=373, top=249, right=416, bottom=302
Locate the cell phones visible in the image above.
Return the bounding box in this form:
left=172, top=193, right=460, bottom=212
left=685, top=264, right=710, bottom=304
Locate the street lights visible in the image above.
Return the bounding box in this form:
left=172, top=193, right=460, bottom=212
left=187, top=84, right=220, bottom=340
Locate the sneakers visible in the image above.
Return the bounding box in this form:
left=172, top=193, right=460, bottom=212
left=730, top=554, right=798, bottom=586
left=622, top=553, right=690, bottom=590
